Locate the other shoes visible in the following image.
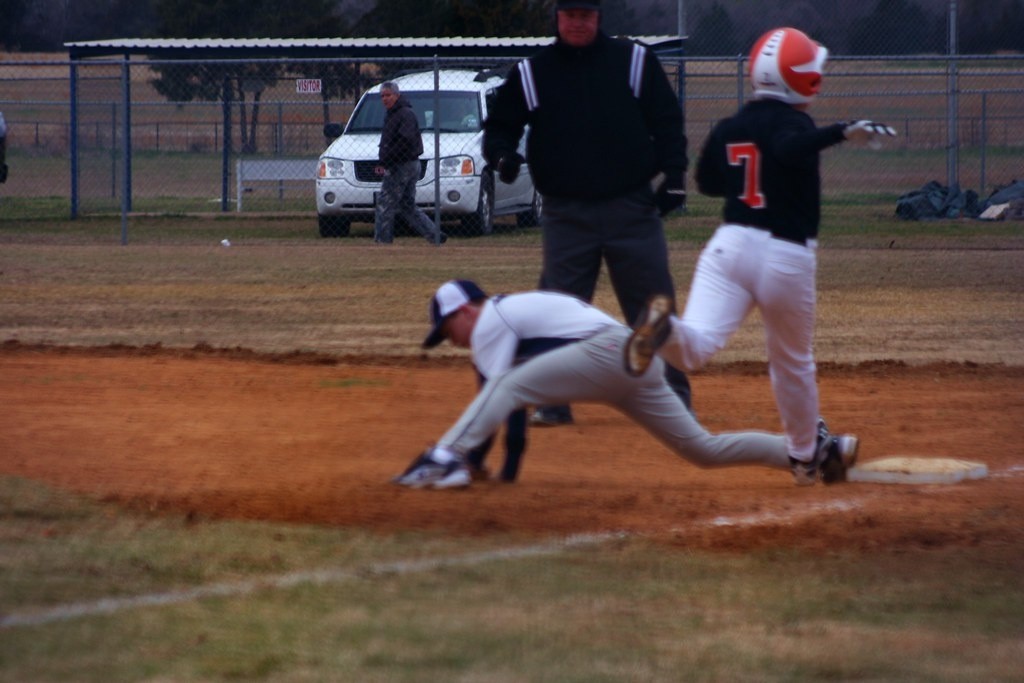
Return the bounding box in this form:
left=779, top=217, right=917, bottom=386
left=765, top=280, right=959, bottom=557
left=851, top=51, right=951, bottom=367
left=528, top=405, right=572, bottom=425
left=428, top=232, right=447, bottom=244
left=665, top=376, right=690, bottom=407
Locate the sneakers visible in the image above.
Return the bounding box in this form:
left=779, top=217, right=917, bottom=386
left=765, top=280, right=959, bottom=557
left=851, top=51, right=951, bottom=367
left=622, top=294, right=671, bottom=376
left=819, top=434, right=857, bottom=485
left=385, top=449, right=471, bottom=490
left=788, top=417, right=829, bottom=486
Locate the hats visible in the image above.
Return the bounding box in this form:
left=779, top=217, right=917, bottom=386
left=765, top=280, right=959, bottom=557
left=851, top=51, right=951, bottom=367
left=553, top=0, right=601, bottom=9
left=421, top=279, right=487, bottom=350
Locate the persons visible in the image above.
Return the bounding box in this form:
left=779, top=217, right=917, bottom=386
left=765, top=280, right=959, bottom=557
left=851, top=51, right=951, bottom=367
left=481, top=0, right=690, bottom=426
left=391, top=279, right=861, bottom=489
left=374, top=82, right=448, bottom=246
left=623, top=27, right=898, bottom=486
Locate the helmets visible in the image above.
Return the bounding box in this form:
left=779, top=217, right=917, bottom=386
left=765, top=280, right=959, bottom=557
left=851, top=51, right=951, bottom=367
left=748, top=26, right=828, bottom=111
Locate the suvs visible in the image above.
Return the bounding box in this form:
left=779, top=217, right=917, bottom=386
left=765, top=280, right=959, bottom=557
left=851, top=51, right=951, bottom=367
left=315, top=67, right=545, bottom=237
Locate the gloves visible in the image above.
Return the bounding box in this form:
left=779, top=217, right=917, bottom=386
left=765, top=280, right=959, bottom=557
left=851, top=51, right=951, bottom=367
left=642, top=170, right=687, bottom=219
left=842, top=119, right=898, bottom=151
left=494, top=154, right=521, bottom=184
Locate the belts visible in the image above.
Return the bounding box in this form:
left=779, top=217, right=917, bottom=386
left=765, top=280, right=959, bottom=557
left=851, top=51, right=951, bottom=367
left=771, top=232, right=806, bottom=247
